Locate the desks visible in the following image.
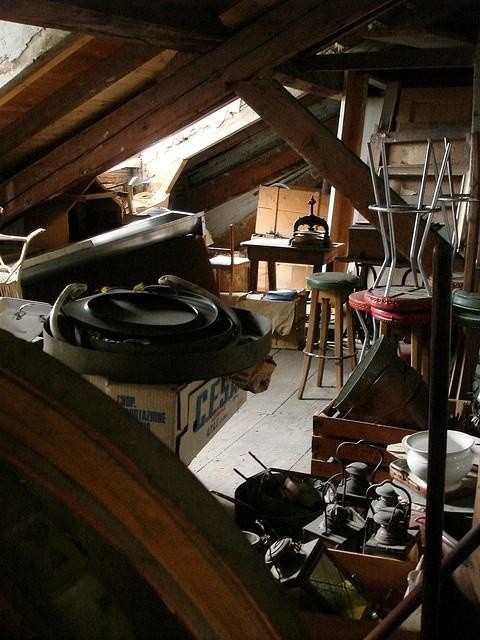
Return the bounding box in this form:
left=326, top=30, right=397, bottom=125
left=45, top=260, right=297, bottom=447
left=240, top=236, right=346, bottom=291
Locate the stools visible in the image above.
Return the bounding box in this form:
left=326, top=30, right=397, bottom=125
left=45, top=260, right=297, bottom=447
left=298, top=272, right=359, bottom=399
left=449, top=312, right=480, bottom=400
left=370, top=308, right=431, bottom=371
left=426, top=132, right=474, bottom=288
left=451, top=129, right=480, bottom=311
left=364, top=139, right=452, bottom=312
left=349, top=289, right=376, bottom=365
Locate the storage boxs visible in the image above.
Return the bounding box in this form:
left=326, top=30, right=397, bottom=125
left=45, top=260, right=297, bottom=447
left=348, top=221, right=387, bottom=260
left=84, top=374, right=248, bottom=465
left=242, top=290, right=310, bottom=349
left=311, top=395, right=471, bottom=481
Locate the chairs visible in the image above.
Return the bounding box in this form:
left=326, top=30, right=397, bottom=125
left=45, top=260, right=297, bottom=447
left=206, top=224, right=250, bottom=303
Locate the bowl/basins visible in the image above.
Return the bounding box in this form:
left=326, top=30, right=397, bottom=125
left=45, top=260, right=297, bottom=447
left=404, top=430, right=475, bottom=492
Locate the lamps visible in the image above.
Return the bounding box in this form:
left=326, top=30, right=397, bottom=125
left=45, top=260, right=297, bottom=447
left=258, top=519, right=373, bottom=622
left=366, top=477, right=408, bottom=547
left=366, top=502, right=422, bottom=566
left=301, top=472, right=364, bottom=553
left=335, top=440, right=383, bottom=517
